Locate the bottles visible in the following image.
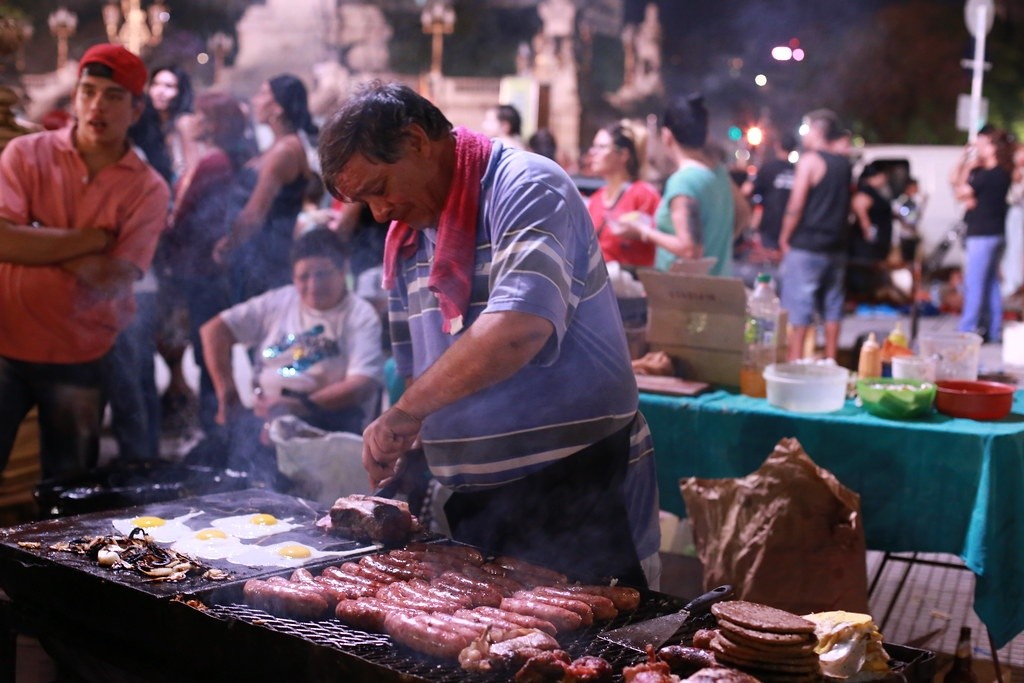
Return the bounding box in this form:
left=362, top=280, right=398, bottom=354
left=739, top=274, right=782, bottom=397
left=942, top=626, right=978, bottom=683
left=857, top=333, right=882, bottom=383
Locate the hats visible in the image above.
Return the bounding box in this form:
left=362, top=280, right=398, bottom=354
left=78, top=44, right=147, bottom=97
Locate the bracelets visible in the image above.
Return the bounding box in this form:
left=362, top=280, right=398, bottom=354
left=640, top=225, right=651, bottom=243
left=281, top=388, right=322, bottom=419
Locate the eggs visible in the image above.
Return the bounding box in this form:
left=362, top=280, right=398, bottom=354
left=209, top=512, right=292, bottom=540
left=227, top=540, right=335, bottom=567
left=170, top=527, right=244, bottom=560
left=112, top=515, right=194, bottom=543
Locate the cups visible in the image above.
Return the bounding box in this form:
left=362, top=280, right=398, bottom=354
left=922, top=331, right=983, bottom=384
left=892, top=354, right=937, bottom=382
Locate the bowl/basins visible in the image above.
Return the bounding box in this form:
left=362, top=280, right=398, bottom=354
left=761, top=362, right=848, bottom=413
left=934, top=379, right=1015, bottom=421
left=858, top=377, right=937, bottom=419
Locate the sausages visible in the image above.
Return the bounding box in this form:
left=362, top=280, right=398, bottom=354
left=658, top=646, right=719, bottom=672
left=244, top=542, right=643, bottom=658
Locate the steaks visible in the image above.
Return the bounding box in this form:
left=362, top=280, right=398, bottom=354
left=709, top=598, right=823, bottom=683
left=330, top=493, right=410, bottom=540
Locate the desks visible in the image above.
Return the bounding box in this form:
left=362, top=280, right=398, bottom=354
left=638, top=386, right=1024, bottom=650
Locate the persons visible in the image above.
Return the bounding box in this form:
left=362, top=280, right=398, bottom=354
left=318, top=78, right=663, bottom=602
left=586, top=120, right=663, bottom=267
left=613, top=90, right=735, bottom=293
left=739, top=109, right=1023, bottom=365
left=527, top=128, right=556, bottom=159
left=1, top=60, right=392, bottom=443
left=200, top=228, right=385, bottom=490
left=0, top=42, right=172, bottom=500
left=483, top=105, right=526, bottom=152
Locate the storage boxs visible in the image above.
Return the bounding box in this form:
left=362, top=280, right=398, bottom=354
left=639, top=259, right=793, bottom=392
